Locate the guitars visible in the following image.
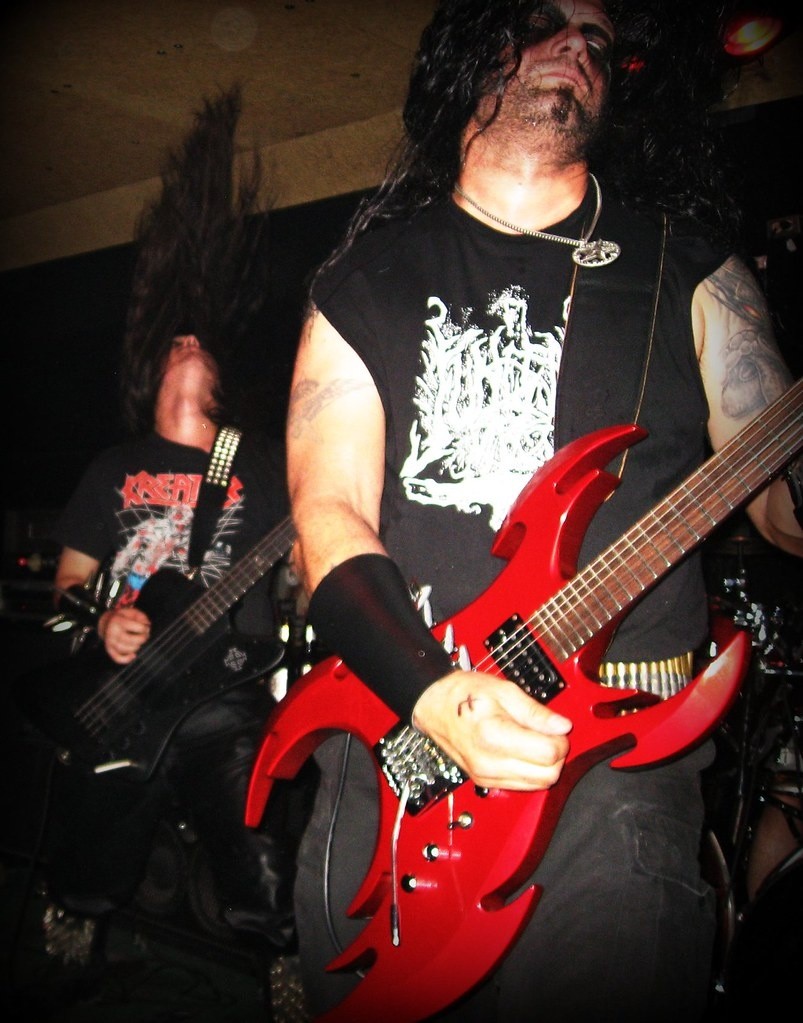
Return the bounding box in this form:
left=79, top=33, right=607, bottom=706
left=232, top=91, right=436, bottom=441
left=10, top=517, right=297, bottom=785
left=244, top=377, right=803, bottom=1023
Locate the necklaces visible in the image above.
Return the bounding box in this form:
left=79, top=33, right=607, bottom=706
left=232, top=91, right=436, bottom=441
left=454, top=171, right=621, bottom=267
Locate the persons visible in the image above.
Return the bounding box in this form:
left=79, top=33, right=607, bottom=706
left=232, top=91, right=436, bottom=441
left=32, top=85, right=313, bottom=1023
left=282, top=0, right=803, bottom=1023
